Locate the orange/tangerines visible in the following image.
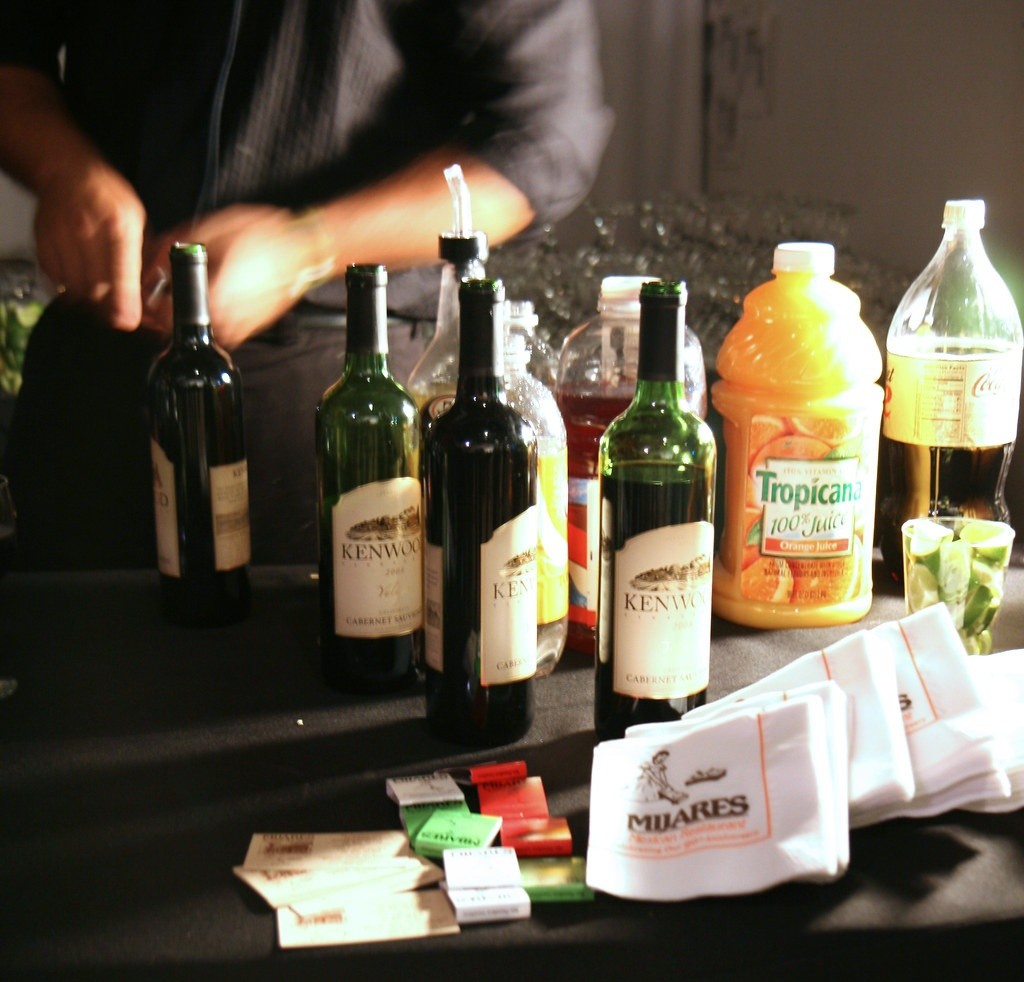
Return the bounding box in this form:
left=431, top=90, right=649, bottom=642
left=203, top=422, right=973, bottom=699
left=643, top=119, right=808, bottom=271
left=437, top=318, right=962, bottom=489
left=743, top=415, right=861, bottom=606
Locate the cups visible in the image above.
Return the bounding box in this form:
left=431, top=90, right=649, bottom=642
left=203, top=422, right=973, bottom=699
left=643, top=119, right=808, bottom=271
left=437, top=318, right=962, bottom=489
left=898, top=516, right=1014, bottom=655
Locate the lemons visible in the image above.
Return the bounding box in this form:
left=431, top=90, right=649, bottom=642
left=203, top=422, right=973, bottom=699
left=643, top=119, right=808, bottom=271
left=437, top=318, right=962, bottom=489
left=905, top=519, right=1012, bottom=656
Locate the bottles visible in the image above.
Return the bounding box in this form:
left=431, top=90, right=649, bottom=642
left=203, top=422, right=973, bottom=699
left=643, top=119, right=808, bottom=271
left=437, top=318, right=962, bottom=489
left=313, top=263, right=424, bottom=696
left=139, top=242, right=253, bottom=629
left=594, top=284, right=717, bottom=740
left=404, top=231, right=569, bottom=747
left=710, top=240, right=886, bottom=627
left=558, top=274, right=708, bottom=659
left=879, top=198, right=1024, bottom=586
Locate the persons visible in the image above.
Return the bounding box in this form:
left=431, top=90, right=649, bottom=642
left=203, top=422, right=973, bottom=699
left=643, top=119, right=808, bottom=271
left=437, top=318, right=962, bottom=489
left=0, top=0, right=612, bottom=579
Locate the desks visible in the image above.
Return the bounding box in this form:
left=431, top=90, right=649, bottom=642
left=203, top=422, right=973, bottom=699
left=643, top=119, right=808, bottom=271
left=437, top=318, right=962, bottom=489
left=1, top=558, right=1024, bottom=982
